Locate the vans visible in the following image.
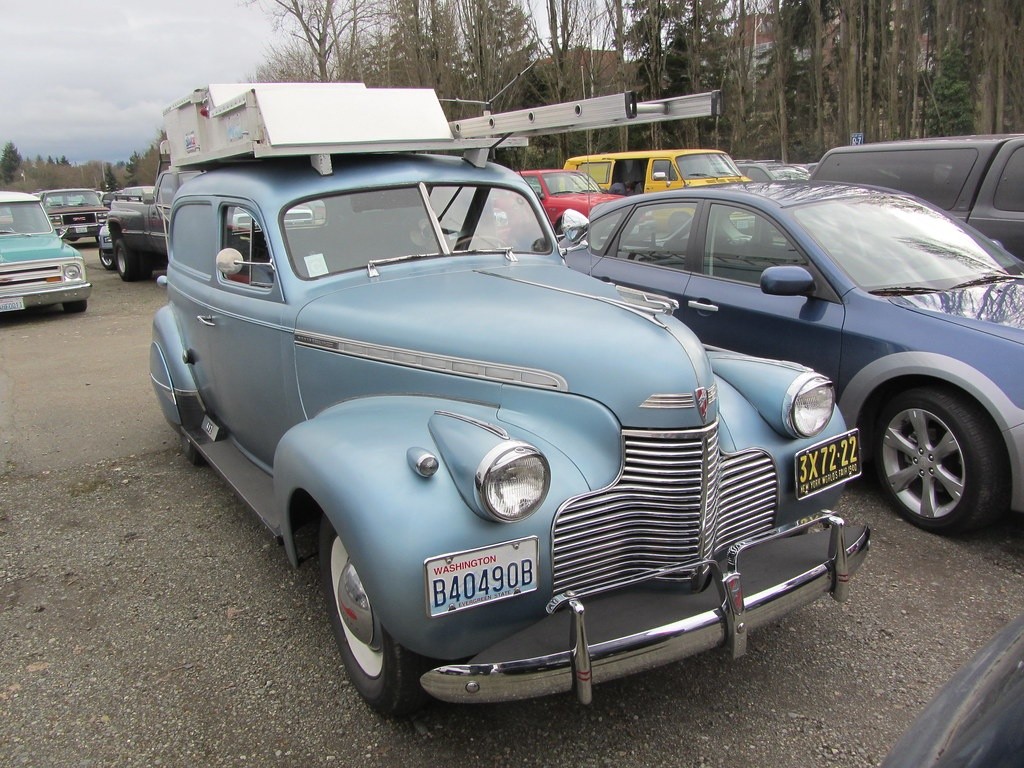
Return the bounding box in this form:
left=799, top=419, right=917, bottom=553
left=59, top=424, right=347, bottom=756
left=561, top=149, right=757, bottom=235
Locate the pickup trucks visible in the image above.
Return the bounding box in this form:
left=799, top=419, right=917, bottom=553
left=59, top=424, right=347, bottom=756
left=810, top=133, right=1024, bottom=266
left=108, top=168, right=315, bottom=284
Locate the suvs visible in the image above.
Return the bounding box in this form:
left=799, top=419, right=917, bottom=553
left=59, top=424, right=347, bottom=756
left=38, top=190, right=111, bottom=244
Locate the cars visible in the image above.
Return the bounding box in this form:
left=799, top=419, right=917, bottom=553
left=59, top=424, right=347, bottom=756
left=734, top=159, right=809, bottom=181
left=558, top=179, right=1023, bottom=537
left=149, top=154, right=872, bottom=721
left=98, top=212, right=116, bottom=270
left=0, top=190, right=92, bottom=315
left=121, top=185, right=157, bottom=200
left=500, top=168, right=628, bottom=243
